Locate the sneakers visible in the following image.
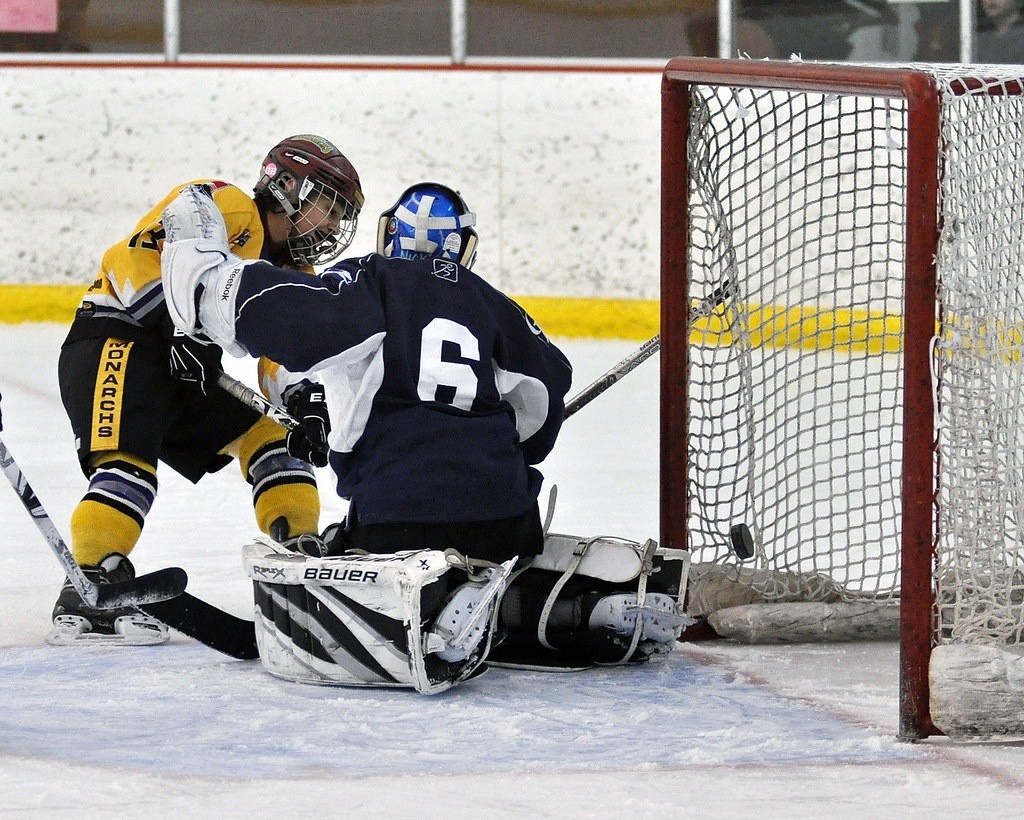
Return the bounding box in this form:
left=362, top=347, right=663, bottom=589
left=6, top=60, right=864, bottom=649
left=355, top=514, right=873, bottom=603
left=269, top=515, right=340, bottom=558
left=431, top=555, right=519, bottom=662
left=546, top=589, right=698, bottom=643
left=46, top=552, right=170, bottom=645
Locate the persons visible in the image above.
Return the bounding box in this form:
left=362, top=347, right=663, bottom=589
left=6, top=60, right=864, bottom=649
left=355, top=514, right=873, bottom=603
left=47, top=134, right=366, bottom=645
left=161, top=181, right=690, bottom=697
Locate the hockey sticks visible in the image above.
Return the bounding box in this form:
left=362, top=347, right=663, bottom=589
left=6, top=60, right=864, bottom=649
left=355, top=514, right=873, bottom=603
left=0, top=436, right=189, bottom=609
left=216, top=372, right=559, bottom=540
left=130, top=276, right=739, bottom=661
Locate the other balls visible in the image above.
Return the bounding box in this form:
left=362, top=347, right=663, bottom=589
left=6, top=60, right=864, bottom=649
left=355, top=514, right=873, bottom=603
left=730, top=522, right=755, bottom=560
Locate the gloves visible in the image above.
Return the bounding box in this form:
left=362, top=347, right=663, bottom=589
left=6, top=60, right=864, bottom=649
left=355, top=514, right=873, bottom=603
left=160, top=333, right=223, bottom=397
left=280, top=378, right=331, bottom=467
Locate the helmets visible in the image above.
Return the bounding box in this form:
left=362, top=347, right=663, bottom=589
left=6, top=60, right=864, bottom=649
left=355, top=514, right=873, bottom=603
left=252, top=134, right=365, bottom=267
left=376, top=183, right=478, bottom=271
